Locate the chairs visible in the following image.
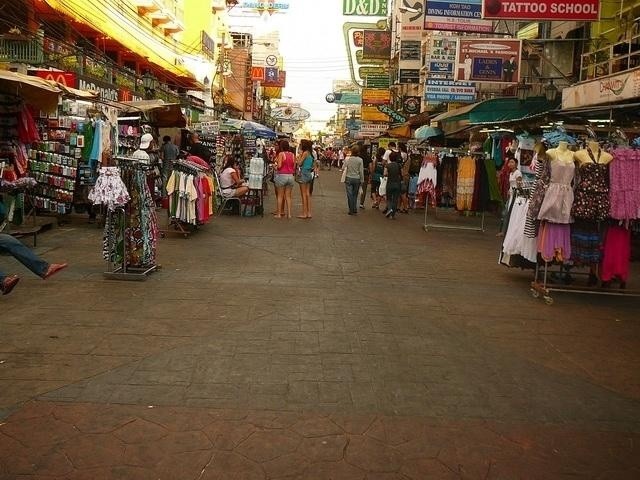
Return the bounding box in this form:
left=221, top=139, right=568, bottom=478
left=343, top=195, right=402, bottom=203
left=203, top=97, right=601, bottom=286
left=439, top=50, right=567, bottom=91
left=214, top=171, right=242, bottom=217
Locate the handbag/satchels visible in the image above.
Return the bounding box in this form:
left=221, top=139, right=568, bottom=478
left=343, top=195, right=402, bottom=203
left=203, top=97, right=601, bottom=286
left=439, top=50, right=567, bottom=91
left=340, top=166, right=347, bottom=182
left=296, top=172, right=312, bottom=183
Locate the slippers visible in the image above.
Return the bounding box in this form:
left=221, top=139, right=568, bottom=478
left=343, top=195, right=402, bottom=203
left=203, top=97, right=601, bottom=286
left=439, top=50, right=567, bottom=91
left=43, top=263, right=68, bottom=280
left=1, top=275, right=20, bottom=295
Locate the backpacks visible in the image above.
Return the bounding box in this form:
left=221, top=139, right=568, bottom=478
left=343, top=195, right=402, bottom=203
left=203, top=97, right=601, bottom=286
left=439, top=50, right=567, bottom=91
left=389, top=147, right=403, bottom=163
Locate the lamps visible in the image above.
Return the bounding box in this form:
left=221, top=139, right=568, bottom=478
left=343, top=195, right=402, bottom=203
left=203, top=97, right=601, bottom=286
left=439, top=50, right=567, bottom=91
left=516, top=77, right=564, bottom=105
left=142, top=70, right=157, bottom=99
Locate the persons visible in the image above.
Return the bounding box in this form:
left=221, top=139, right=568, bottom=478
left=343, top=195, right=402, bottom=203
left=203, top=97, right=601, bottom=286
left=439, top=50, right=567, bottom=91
left=496, top=157, right=523, bottom=237
left=132, top=134, right=154, bottom=183
left=502, top=56, right=520, bottom=82
left=180, top=131, right=212, bottom=165
left=537, top=139, right=577, bottom=225
left=574, top=140, right=612, bottom=223
left=463, top=53, right=473, bottom=80
left=220, top=157, right=249, bottom=199
left=269, top=138, right=412, bottom=219
left=158, top=135, right=180, bottom=198
left=0, top=233, right=68, bottom=295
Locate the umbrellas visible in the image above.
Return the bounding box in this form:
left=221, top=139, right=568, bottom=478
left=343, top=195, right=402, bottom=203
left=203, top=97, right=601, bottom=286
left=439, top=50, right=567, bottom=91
left=415, top=123, right=443, bottom=139
left=386, top=122, right=415, bottom=139
left=223, top=117, right=277, bottom=138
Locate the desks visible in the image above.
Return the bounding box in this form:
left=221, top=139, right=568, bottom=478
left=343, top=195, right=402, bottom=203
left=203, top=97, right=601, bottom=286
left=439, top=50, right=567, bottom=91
left=243, top=186, right=264, bottom=217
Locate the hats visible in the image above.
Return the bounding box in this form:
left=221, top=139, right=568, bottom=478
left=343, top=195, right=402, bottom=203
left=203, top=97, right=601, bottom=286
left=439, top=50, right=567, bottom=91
left=139, top=133, right=153, bottom=149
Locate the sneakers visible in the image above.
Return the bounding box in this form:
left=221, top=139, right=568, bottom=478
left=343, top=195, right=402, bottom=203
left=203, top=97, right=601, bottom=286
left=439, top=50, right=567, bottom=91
left=372, top=201, right=408, bottom=219
